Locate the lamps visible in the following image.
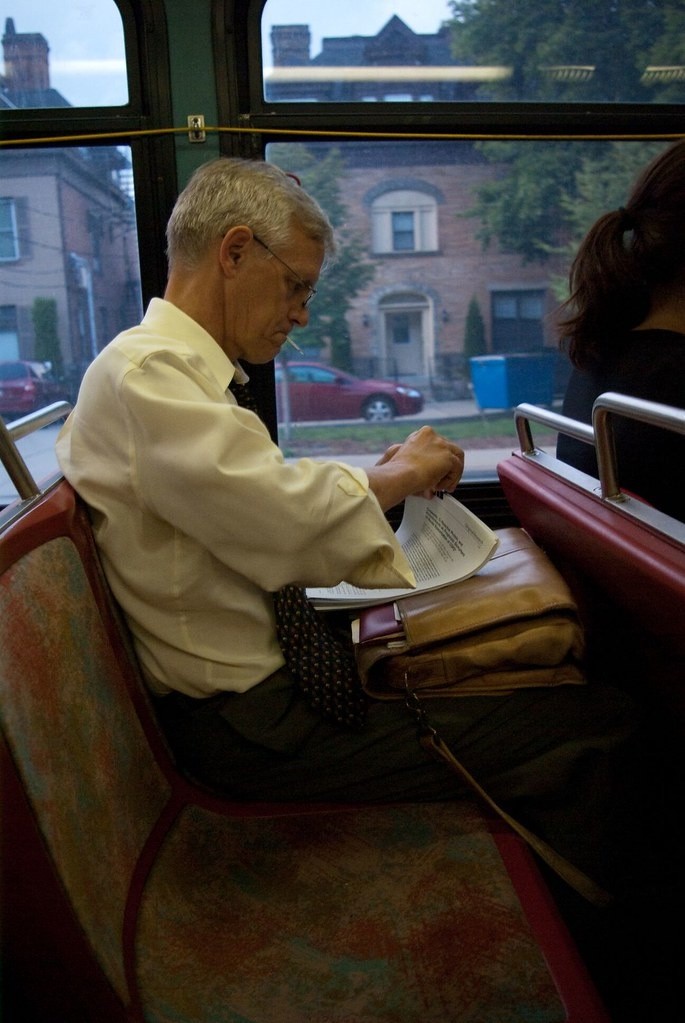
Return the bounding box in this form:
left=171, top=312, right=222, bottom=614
left=361, top=313, right=370, bottom=328
left=441, top=308, right=449, bottom=322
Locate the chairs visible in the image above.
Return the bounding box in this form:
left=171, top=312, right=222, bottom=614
left=0, top=488, right=610, bottom=1023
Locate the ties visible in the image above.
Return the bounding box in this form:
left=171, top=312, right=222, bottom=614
left=228, top=376, right=370, bottom=736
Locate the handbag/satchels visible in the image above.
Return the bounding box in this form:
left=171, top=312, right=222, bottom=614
left=353, top=527, right=587, bottom=700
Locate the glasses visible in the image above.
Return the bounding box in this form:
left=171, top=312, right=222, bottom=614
left=253, top=237, right=318, bottom=308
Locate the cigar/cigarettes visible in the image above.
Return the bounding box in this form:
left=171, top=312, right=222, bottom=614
left=287, top=337, right=304, bottom=356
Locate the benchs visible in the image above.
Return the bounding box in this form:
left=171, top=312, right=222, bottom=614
left=501, top=388, right=685, bottom=657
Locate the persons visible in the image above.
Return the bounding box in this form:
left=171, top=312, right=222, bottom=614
left=52, top=156, right=650, bottom=1023
left=557, top=140, right=685, bottom=517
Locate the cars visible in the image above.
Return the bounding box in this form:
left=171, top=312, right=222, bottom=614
left=274, top=361, right=425, bottom=425
left=0, top=358, right=68, bottom=418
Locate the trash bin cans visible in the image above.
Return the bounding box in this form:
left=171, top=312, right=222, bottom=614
left=468, top=351, right=555, bottom=410
